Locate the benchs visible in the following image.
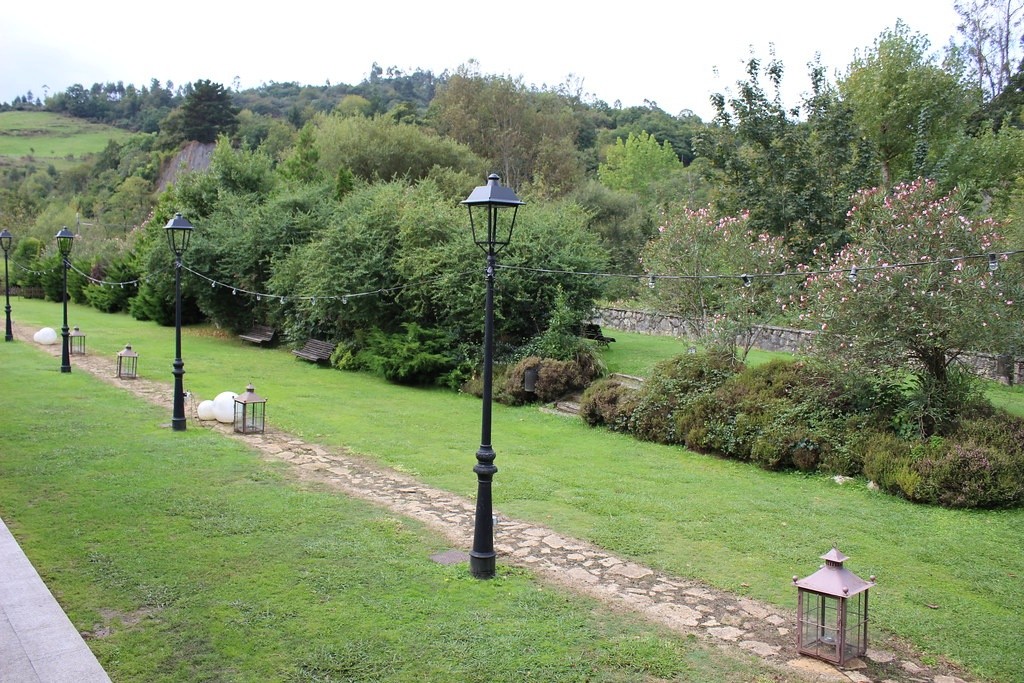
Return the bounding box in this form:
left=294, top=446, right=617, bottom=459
left=239, top=324, right=275, bottom=347
left=291, top=338, right=336, bottom=369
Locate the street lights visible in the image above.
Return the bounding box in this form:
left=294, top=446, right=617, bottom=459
left=0, top=229, right=13, bottom=342
left=461, top=174, right=527, bottom=581
left=162, top=212, right=195, bottom=433
left=55, top=226, right=75, bottom=373
